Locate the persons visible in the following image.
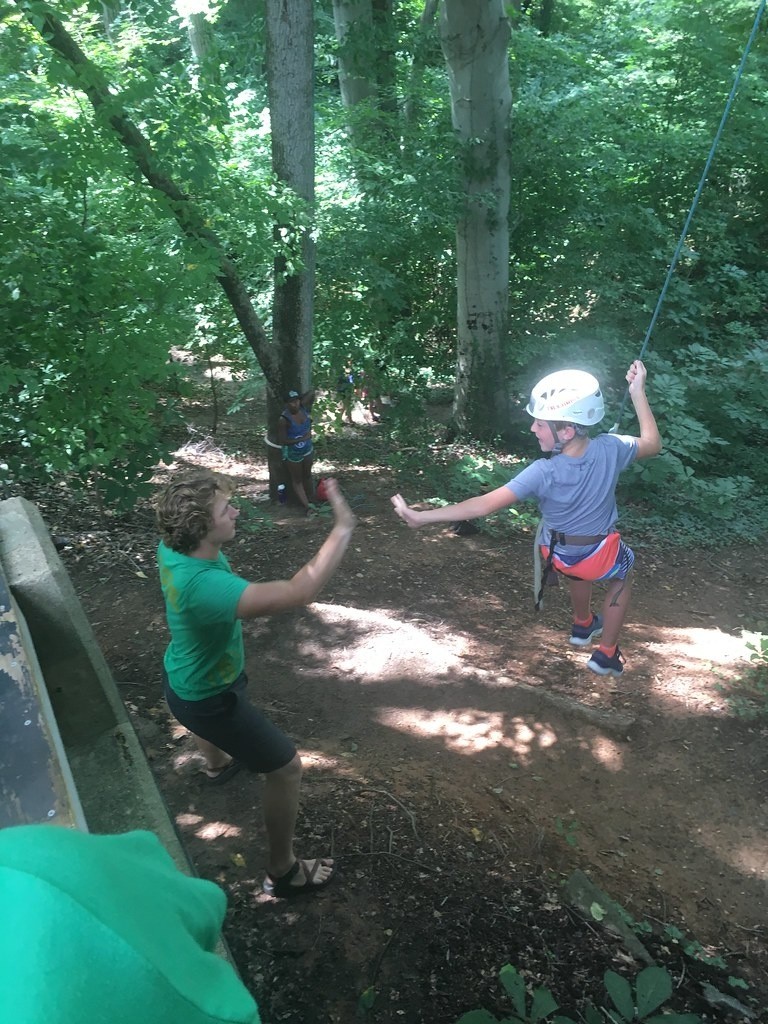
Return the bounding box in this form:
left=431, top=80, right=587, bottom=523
left=389, top=359, right=662, bottom=674
left=360, top=371, right=390, bottom=421
left=156, top=470, right=359, bottom=898
left=335, top=372, right=356, bottom=424
left=277, top=390, right=318, bottom=516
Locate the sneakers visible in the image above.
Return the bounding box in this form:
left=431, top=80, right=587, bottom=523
left=587, top=644, right=627, bottom=675
left=569, top=614, right=603, bottom=646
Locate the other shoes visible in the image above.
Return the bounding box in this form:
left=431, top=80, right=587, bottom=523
left=372, top=417, right=391, bottom=423
left=337, top=422, right=357, bottom=427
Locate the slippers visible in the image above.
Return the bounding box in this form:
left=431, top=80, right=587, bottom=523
left=306, top=507, right=315, bottom=517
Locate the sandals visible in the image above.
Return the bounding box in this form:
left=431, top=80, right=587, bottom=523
left=262, top=857, right=337, bottom=897
left=205, top=755, right=241, bottom=785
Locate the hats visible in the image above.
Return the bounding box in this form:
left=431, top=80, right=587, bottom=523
left=283, top=388, right=303, bottom=402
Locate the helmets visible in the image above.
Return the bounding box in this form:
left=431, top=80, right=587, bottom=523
left=525, top=369, right=605, bottom=426
